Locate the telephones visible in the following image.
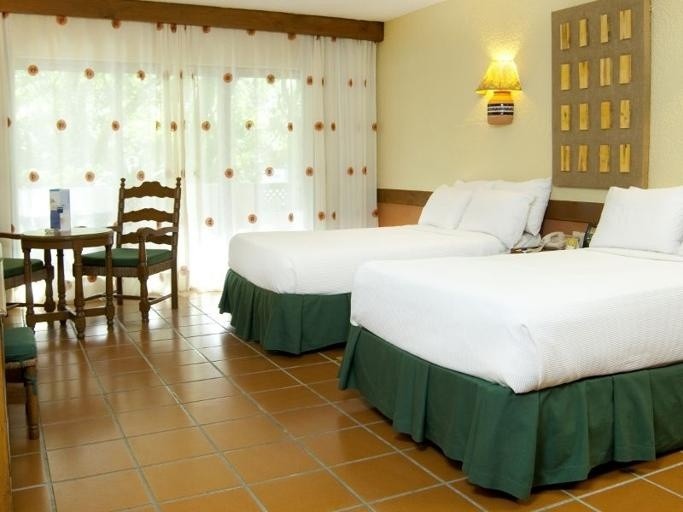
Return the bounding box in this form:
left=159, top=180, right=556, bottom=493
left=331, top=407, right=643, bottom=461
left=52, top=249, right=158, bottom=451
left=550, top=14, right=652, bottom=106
left=539, top=231, right=566, bottom=250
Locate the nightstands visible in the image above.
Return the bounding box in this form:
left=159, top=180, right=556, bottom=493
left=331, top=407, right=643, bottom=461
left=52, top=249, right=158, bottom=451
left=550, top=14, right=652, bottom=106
left=509, top=244, right=565, bottom=255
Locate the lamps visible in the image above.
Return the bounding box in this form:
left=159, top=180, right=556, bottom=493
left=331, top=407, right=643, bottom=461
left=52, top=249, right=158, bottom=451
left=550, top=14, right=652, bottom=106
left=473, top=47, right=523, bottom=129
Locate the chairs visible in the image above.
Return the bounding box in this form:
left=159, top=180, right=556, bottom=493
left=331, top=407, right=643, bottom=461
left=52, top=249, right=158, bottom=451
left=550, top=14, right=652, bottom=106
left=0, top=230, right=56, bottom=327
left=1, top=322, right=39, bottom=448
left=74, top=176, right=182, bottom=330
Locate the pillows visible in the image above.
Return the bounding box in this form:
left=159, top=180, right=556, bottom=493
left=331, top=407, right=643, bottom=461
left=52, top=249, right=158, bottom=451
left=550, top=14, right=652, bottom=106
left=417, top=175, right=555, bottom=247
left=588, top=185, right=683, bottom=254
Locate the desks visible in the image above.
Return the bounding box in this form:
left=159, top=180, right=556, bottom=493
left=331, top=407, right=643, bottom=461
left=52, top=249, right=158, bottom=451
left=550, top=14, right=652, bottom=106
left=19, top=225, right=116, bottom=339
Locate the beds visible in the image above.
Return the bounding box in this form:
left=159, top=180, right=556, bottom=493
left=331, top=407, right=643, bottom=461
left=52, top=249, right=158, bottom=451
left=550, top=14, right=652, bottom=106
left=336, top=247, right=683, bottom=496
left=217, top=227, right=552, bottom=359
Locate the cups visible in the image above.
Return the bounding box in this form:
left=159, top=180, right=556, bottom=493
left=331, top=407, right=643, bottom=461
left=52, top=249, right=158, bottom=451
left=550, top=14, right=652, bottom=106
left=565, top=235, right=579, bottom=249
